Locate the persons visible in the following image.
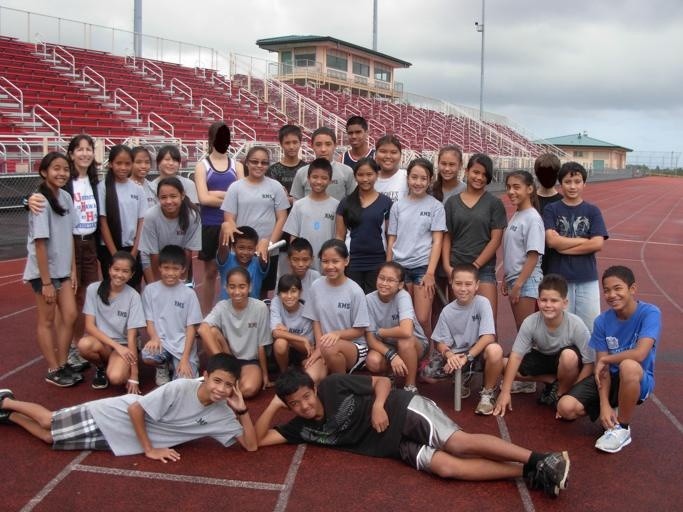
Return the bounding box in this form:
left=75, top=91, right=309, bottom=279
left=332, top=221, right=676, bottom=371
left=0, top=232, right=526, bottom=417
left=270, top=273, right=314, bottom=382
left=147, top=146, right=201, bottom=204
left=372, top=135, right=408, bottom=253
left=194, top=123, right=244, bottom=315
left=431, top=146, right=468, bottom=207
left=304, top=238, right=371, bottom=383
left=196, top=266, right=275, bottom=397
left=24, top=135, right=100, bottom=371
left=288, top=128, right=357, bottom=205
left=254, top=367, right=570, bottom=499
left=97, top=145, right=149, bottom=293
left=365, top=262, right=429, bottom=394
left=341, top=117, right=377, bottom=175
left=130, top=146, right=156, bottom=208
left=420, top=263, right=504, bottom=415
left=499, top=170, right=546, bottom=393
left=443, top=153, right=508, bottom=343
left=544, top=162, right=610, bottom=334
left=534, top=153, right=564, bottom=212
left=0, top=352, right=258, bottom=464
left=77, top=251, right=148, bottom=395
left=221, top=146, right=291, bottom=298
left=336, top=158, right=393, bottom=297
left=141, top=245, right=202, bottom=385
left=557, top=266, right=661, bottom=454
left=386, top=159, right=448, bottom=382
left=21, top=151, right=85, bottom=387
left=494, top=275, right=597, bottom=418
left=265, top=124, right=310, bottom=198
left=138, top=178, right=202, bottom=285
left=275, top=238, right=322, bottom=305
left=282, top=158, right=341, bottom=271
left=216, top=222, right=269, bottom=302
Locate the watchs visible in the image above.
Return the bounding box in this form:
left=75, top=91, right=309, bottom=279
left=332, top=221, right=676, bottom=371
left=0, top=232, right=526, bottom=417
left=235, top=409, right=249, bottom=417
left=464, top=352, right=474, bottom=362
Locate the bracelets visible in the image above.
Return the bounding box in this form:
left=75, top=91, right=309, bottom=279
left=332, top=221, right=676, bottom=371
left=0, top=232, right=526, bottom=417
left=41, top=283, right=53, bottom=287
left=128, top=380, right=139, bottom=384
left=385, top=350, right=397, bottom=361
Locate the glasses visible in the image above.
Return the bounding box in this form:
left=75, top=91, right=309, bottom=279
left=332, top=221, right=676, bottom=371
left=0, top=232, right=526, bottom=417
left=248, top=158, right=269, bottom=165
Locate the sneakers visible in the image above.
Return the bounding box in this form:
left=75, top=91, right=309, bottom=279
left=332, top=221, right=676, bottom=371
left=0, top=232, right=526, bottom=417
left=595, top=425, right=632, bottom=453
left=61, top=364, right=85, bottom=381
left=74, top=347, right=91, bottom=369
left=404, top=385, right=418, bottom=394
left=461, top=371, right=471, bottom=398
left=92, top=367, right=109, bottom=389
left=45, top=369, right=76, bottom=387
left=524, top=467, right=559, bottom=498
left=537, top=383, right=551, bottom=404
left=500, top=380, right=536, bottom=393
left=545, top=380, right=559, bottom=405
left=156, top=364, right=169, bottom=386
left=1, top=410, right=10, bottom=422
left=68, top=352, right=84, bottom=372
left=474, top=386, right=499, bottom=415
left=537, top=450, right=570, bottom=489
left=0, top=389, right=13, bottom=401
left=387, top=374, right=395, bottom=384
